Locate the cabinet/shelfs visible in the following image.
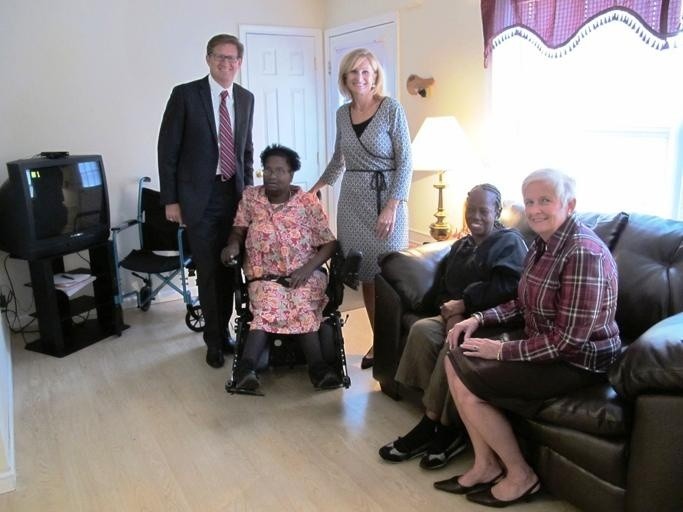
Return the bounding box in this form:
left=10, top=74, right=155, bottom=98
left=22, top=238, right=130, bottom=359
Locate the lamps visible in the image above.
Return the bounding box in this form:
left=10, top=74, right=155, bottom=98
left=411, top=116, right=466, bottom=242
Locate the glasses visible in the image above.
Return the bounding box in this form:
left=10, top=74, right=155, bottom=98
left=211, top=53, right=239, bottom=62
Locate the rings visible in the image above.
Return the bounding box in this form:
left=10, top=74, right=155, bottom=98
left=384, top=228, right=389, bottom=231
left=448, top=329, right=452, bottom=336
left=472, top=345, right=479, bottom=350
left=169, top=218, right=173, bottom=221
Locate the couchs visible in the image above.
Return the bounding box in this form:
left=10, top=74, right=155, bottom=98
left=370, top=211, right=683, bottom=512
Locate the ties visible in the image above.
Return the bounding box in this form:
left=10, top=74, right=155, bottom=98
left=219, top=91, right=236, bottom=180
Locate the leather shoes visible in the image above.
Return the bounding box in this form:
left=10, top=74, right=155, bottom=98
left=379, top=431, right=437, bottom=462
left=206, top=336, right=236, bottom=368
left=433, top=471, right=506, bottom=494
left=361, top=345, right=374, bottom=369
left=466, top=477, right=541, bottom=508
left=419, top=434, right=467, bottom=470
left=232, top=358, right=261, bottom=389
left=309, top=362, right=340, bottom=387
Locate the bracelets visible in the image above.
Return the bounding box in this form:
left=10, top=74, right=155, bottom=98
left=470, top=313, right=481, bottom=323
left=497, top=348, right=500, bottom=361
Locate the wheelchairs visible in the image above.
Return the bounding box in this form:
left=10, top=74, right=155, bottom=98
left=226, top=236, right=363, bottom=395
left=72, top=195, right=104, bottom=230
left=110, top=174, right=211, bottom=331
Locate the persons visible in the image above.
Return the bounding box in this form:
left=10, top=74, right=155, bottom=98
left=377, top=182, right=529, bottom=470
left=157, top=34, right=255, bottom=367
left=221, top=144, right=342, bottom=392
left=434, top=168, right=623, bottom=509
left=304, top=47, right=413, bottom=369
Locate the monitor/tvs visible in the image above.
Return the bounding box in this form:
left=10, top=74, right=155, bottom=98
left=0, top=154, right=111, bottom=260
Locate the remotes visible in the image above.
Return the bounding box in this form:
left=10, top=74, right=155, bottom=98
left=40, top=151, right=69, bottom=157
